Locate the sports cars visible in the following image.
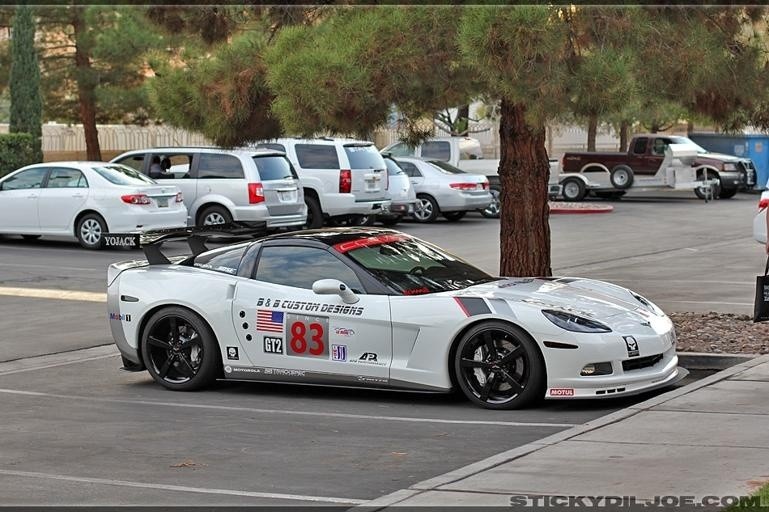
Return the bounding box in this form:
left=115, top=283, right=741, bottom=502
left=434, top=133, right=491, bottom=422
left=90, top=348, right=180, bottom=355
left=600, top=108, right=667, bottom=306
left=107, top=226, right=690, bottom=410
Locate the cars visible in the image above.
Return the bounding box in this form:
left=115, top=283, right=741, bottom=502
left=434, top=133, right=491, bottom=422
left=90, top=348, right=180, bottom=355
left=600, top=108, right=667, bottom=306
left=0, top=134, right=769, bottom=250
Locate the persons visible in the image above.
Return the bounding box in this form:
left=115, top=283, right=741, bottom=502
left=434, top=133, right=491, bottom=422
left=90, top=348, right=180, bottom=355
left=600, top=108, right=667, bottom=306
left=151, top=155, right=162, bottom=170
